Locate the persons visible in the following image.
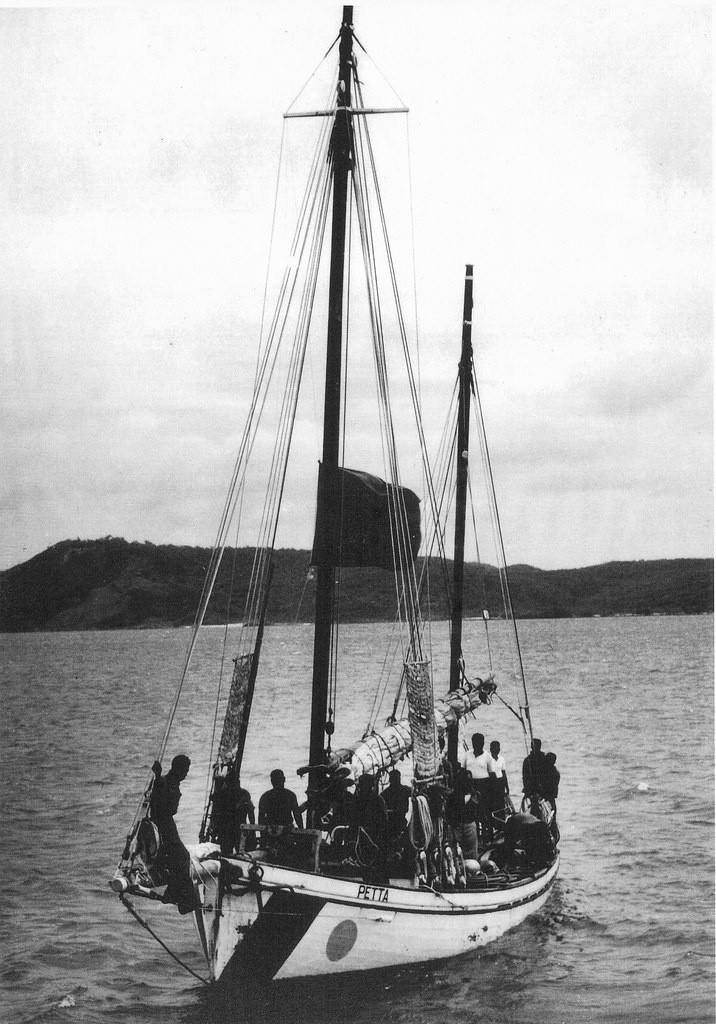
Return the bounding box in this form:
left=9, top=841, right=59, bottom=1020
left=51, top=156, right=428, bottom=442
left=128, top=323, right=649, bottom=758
left=149, top=733, right=560, bottom=874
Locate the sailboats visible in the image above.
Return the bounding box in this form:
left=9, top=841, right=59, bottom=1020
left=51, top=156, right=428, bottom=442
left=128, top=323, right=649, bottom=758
left=102, top=2, right=565, bottom=1001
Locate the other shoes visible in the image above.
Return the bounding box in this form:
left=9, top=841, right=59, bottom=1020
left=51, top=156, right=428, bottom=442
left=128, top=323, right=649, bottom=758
left=162, top=890, right=182, bottom=902
left=178, top=903, right=203, bottom=914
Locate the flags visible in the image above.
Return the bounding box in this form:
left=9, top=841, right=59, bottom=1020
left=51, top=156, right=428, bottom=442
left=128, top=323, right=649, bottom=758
left=312, top=465, right=421, bottom=569
